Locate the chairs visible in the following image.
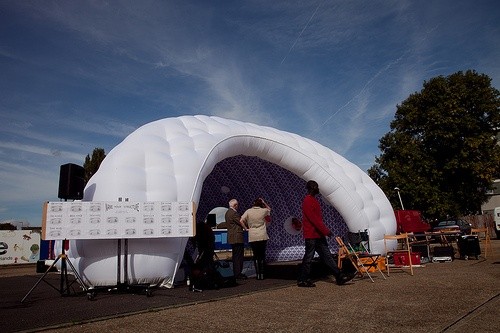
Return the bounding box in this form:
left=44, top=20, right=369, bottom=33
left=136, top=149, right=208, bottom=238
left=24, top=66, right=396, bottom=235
left=335, top=227, right=494, bottom=283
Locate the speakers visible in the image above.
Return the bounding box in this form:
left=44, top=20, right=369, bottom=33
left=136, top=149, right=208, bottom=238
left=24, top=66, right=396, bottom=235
left=207, top=214, right=216, bottom=227
left=434, top=246, right=454, bottom=260
left=456, top=235, right=481, bottom=256
left=58, top=163, right=85, bottom=200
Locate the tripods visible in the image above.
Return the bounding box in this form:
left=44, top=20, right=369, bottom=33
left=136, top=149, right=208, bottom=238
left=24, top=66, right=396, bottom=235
left=21, top=199, right=88, bottom=305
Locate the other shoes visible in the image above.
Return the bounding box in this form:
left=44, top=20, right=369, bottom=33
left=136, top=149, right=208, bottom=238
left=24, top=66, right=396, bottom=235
left=260, top=274, right=264, bottom=280
left=297, top=280, right=316, bottom=287
left=257, top=274, right=260, bottom=280
left=338, top=275, right=353, bottom=285
left=236, top=274, right=247, bottom=279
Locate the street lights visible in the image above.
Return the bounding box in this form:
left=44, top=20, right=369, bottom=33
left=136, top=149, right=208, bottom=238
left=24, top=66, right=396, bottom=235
left=394, top=187, right=405, bottom=210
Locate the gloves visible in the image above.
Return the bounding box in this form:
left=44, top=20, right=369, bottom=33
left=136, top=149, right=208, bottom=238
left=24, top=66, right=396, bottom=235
left=328, top=231, right=334, bottom=237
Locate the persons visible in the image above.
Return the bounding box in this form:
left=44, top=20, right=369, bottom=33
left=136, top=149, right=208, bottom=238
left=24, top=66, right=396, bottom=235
left=295, top=180, right=356, bottom=287
left=21, top=244, right=39, bottom=262
left=240, top=197, right=272, bottom=280
left=225, top=198, right=247, bottom=280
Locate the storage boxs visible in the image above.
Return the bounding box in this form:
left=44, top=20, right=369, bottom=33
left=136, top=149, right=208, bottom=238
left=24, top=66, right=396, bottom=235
left=392, top=251, right=421, bottom=266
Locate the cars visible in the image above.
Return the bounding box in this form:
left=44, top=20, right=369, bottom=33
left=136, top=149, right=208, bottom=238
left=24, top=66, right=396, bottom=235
left=432, top=219, right=472, bottom=237
